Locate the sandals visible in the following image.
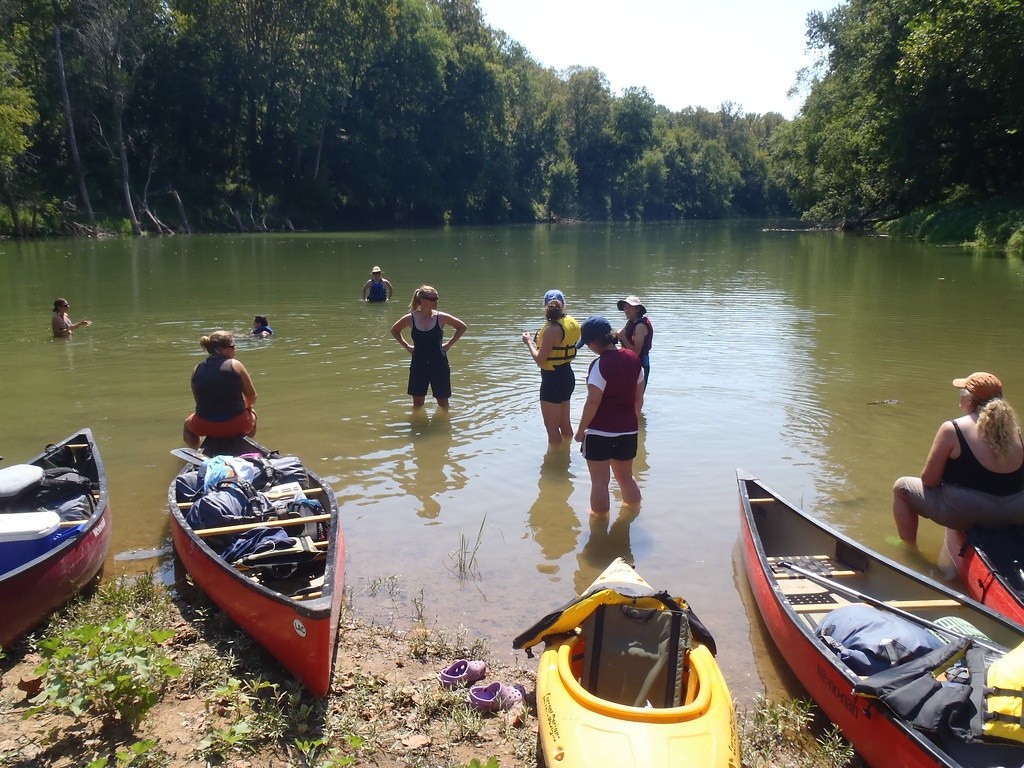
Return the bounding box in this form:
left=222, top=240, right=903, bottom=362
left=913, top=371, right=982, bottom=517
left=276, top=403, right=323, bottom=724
left=468, top=681, right=527, bottom=713
left=438, top=659, right=486, bottom=689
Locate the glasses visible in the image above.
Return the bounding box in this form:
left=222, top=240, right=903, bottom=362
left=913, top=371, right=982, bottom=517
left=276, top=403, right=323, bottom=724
left=220, top=344, right=236, bottom=349
left=419, top=296, right=439, bottom=302
left=59, top=304, right=68, bottom=308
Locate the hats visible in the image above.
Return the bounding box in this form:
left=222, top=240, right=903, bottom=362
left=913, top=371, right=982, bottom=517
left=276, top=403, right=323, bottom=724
left=952, top=371, right=1004, bottom=401
left=544, top=289, right=564, bottom=304
left=371, top=266, right=382, bottom=273
left=617, top=295, right=646, bottom=315
left=575, top=316, right=611, bottom=349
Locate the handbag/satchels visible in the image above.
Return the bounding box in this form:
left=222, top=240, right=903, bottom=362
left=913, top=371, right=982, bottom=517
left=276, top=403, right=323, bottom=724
left=19, top=442, right=97, bottom=521
left=175, top=452, right=322, bottom=574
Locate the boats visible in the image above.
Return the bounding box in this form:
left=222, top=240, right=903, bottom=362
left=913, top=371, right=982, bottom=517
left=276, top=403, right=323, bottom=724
left=944, top=527, right=1024, bottom=628
left=0, top=428, right=112, bottom=649
left=167, top=436, right=346, bottom=697
left=513, top=557, right=742, bottom=768
left=737, top=469, right=1024, bottom=768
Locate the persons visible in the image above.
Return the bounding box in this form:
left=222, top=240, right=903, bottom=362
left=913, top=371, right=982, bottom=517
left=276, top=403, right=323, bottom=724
left=522, top=289, right=581, bottom=444
left=253, top=315, right=273, bottom=337
left=182, top=331, right=258, bottom=448
left=391, top=285, right=467, bottom=407
left=891, top=372, right=1024, bottom=543
left=362, top=266, right=393, bottom=303
left=51, top=298, right=92, bottom=336
left=615, top=295, right=653, bottom=390
left=573, top=315, right=646, bottom=513
